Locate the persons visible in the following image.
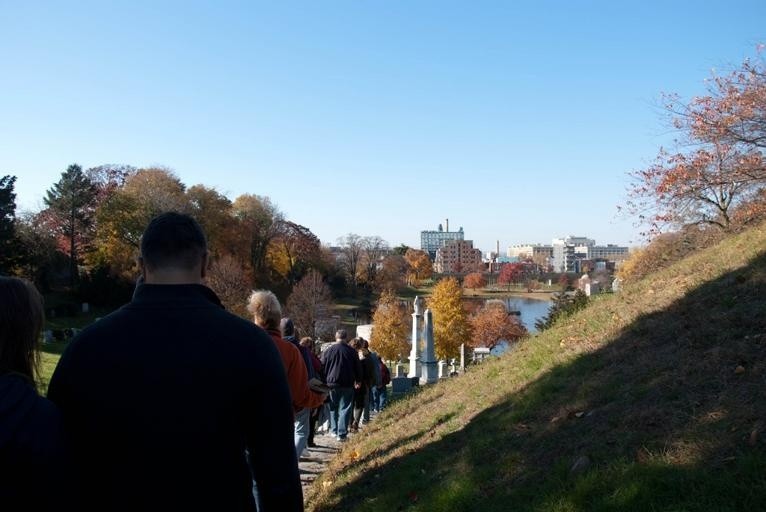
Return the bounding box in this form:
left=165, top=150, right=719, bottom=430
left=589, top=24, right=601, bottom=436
left=0, top=272, right=47, bottom=511
left=247, top=288, right=394, bottom=465
left=17, top=210, right=307, bottom=511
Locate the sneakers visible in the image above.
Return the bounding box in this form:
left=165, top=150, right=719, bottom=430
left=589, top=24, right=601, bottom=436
left=300, top=447, right=310, bottom=457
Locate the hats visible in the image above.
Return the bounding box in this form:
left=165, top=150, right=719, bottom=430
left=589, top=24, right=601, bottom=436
left=335, top=330, right=347, bottom=338
left=281, top=317, right=292, bottom=332
